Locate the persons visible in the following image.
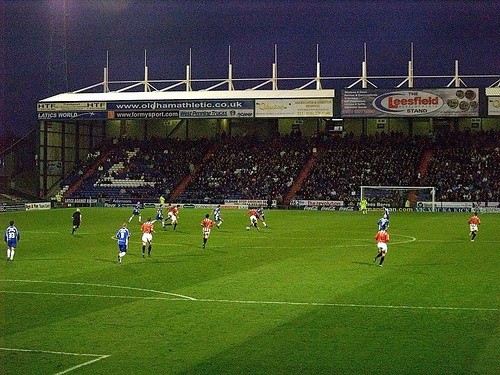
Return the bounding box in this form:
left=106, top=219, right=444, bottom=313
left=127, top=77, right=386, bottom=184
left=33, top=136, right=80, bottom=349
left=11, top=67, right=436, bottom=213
left=140, top=217, right=157, bottom=257
left=71, top=208, right=82, bottom=235
left=467, top=212, right=482, bottom=242
left=359, top=197, right=368, bottom=215
left=374, top=226, right=389, bottom=266
left=0, top=126, right=500, bottom=215
left=4, top=220, right=20, bottom=261
left=209, top=205, right=224, bottom=229
left=244, top=207, right=268, bottom=230
left=116, top=222, right=130, bottom=265
left=127, top=201, right=143, bottom=224
left=200, top=214, right=215, bottom=249
left=151, top=203, right=181, bottom=231
left=377, top=205, right=391, bottom=231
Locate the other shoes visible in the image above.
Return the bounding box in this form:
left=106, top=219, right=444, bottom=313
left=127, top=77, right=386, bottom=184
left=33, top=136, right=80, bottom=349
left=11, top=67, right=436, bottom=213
left=216, top=224, right=220, bottom=230
left=471, top=240, right=474, bottom=242
left=118, top=255, right=121, bottom=263
left=469, top=232, right=472, bottom=235
left=142, top=253, right=145, bottom=258
left=379, top=265, right=383, bottom=267
left=148, top=254, right=151, bottom=257
left=373, top=257, right=376, bottom=262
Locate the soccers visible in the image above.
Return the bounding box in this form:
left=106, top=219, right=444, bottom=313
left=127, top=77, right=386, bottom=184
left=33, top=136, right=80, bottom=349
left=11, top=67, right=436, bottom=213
left=246, top=226, right=251, bottom=230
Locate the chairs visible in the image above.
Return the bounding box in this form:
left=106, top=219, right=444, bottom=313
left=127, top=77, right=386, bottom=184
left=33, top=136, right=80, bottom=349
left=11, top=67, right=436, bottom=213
left=50, top=141, right=249, bottom=204
